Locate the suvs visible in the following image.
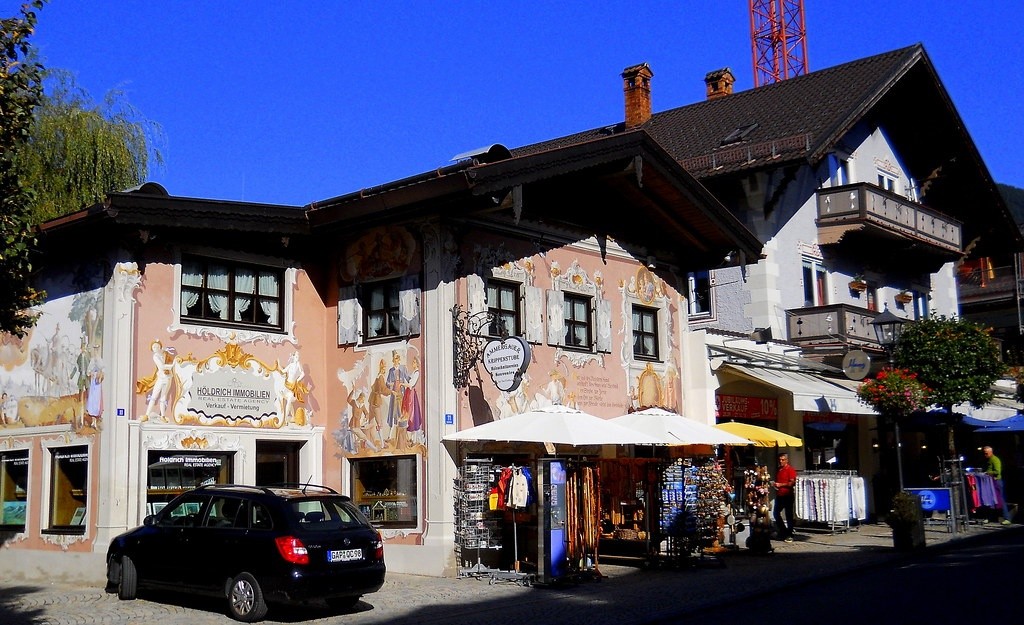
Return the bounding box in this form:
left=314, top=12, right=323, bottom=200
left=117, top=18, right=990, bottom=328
left=106, top=482, right=387, bottom=623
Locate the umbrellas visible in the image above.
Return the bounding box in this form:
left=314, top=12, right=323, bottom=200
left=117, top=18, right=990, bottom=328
left=443, top=402, right=803, bottom=447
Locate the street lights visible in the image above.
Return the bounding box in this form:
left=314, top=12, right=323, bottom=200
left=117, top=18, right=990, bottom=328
left=868, top=300, right=906, bottom=494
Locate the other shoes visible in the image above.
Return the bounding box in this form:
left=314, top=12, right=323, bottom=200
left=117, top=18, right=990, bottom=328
left=1001, top=520, right=1012, bottom=525
left=982, top=519, right=990, bottom=523
left=785, top=537, right=794, bottom=543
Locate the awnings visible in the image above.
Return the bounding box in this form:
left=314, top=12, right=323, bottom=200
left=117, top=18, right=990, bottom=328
left=724, top=363, right=1024, bottom=432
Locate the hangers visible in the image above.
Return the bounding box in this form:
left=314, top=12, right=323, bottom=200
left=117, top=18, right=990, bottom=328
left=803, top=469, right=858, bottom=478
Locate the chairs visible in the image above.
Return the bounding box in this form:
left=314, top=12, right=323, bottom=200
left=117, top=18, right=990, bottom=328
left=221, top=501, right=250, bottom=528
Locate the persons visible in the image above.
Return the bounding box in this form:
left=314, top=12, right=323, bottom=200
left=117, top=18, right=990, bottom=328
left=984, top=445, right=1012, bottom=525
left=770, top=453, right=797, bottom=543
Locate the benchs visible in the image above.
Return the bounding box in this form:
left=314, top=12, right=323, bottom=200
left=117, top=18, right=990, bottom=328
left=294, top=512, right=329, bottom=530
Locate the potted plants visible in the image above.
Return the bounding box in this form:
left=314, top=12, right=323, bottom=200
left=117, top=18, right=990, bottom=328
left=849, top=272, right=869, bottom=297
left=894, top=284, right=914, bottom=307
